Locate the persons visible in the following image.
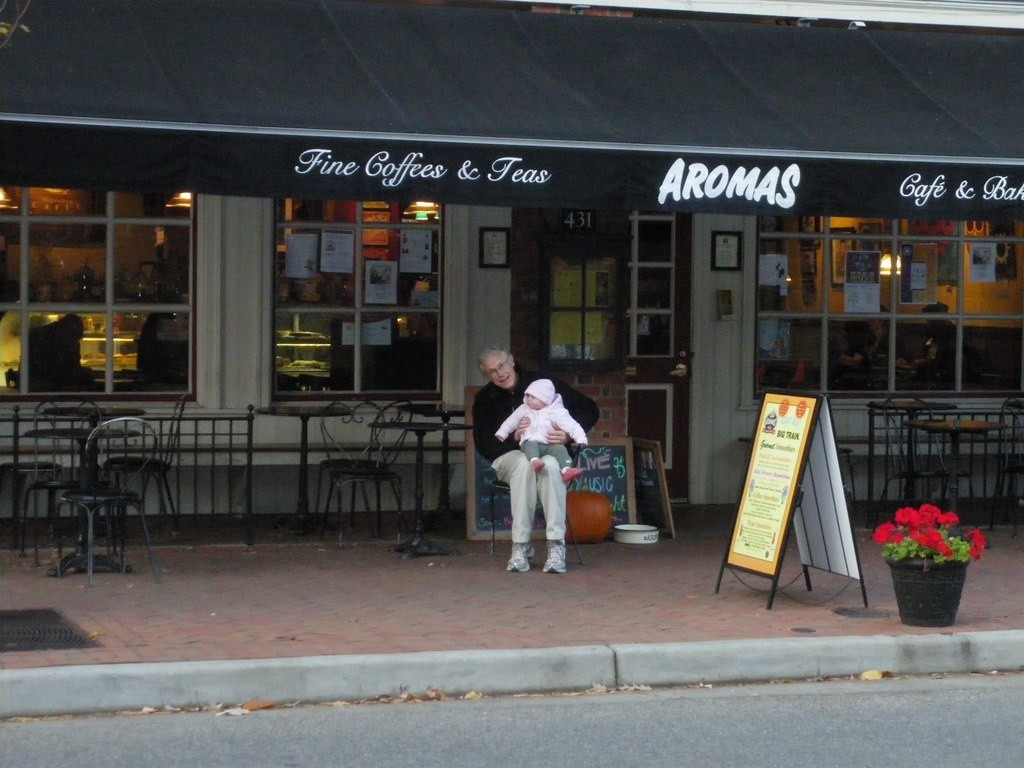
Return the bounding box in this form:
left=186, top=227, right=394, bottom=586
left=472, top=343, right=600, bottom=573
left=137, top=259, right=197, bottom=390
left=866, top=303, right=981, bottom=391
left=283, top=262, right=438, bottom=391
left=494, top=379, right=588, bottom=482
left=19, top=314, right=95, bottom=393
left=594, top=267, right=690, bottom=361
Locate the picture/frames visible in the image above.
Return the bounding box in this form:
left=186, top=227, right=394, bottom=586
left=478, top=226, right=511, bottom=269
left=361, top=207, right=391, bottom=248
left=857, top=222, right=882, bottom=251
left=711, top=230, right=742, bottom=273
left=830, top=227, right=856, bottom=289
left=798, top=216, right=821, bottom=247
left=799, top=248, right=818, bottom=275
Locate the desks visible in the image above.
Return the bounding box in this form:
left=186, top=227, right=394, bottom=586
left=874, top=399, right=957, bottom=514
left=397, top=402, right=465, bottom=533
left=903, top=418, right=1012, bottom=526
left=255, top=405, right=353, bottom=538
left=24, top=426, right=141, bottom=578
left=844, top=366, right=918, bottom=389
left=368, top=419, right=476, bottom=561
left=40, top=403, right=146, bottom=540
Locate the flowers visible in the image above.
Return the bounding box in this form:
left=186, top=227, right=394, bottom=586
left=870, top=505, right=988, bottom=564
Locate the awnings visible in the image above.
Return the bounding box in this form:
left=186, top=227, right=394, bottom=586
left=0, top=0, right=1024, bottom=224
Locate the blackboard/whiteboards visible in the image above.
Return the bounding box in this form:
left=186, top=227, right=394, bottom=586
left=633, top=438, right=674, bottom=533
left=466, top=436, right=636, bottom=541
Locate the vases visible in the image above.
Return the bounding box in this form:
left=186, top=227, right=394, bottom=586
left=883, top=553, right=969, bottom=627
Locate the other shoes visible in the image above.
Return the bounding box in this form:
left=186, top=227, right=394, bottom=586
left=560, top=467, right=581, bottom=481
left=532, top=459, right=545, bottom=472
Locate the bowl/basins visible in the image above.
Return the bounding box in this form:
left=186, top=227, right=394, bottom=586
left=613, top=524, right=660, bottom=545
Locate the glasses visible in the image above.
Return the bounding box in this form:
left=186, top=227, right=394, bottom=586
left=483, top=356, right=509, bottom=378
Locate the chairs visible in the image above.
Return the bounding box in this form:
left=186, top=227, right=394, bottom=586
left=987, top=392, right=1024, bottom=539
left=489, top=443, right=585, bottom=567
left=836, top=445, right=858, bottom=512
left=0, top=397, right=414, bottom=587
left=883, top=394, right=979, bottom=529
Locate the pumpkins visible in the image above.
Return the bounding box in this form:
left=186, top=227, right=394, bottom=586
left=565, top=490, right=612, bottom=544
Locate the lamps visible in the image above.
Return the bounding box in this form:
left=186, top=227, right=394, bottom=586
left=403, top=201, right=438, bottom=215
left=165, top=193, right=191, bottom=207
left=880, top=252, right=901, bottom=276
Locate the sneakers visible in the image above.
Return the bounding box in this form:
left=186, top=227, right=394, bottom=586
left=543, top=540, right=566, bottom=573
left=506, top=541, right=535, bottom=572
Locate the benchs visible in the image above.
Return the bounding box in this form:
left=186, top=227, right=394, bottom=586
left=0, top=440, right=467, bottom=521
left=736, top=434, right=1024, bottom=500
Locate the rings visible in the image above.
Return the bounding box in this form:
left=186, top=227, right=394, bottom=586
left=558, top=440, right=560, bottom=444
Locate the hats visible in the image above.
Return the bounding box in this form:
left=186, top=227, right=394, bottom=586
left=523, top=379, right=555, bottom=406
